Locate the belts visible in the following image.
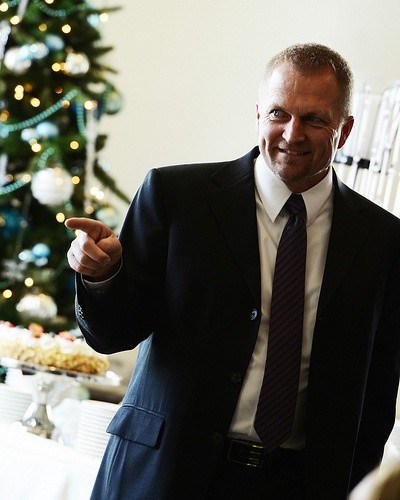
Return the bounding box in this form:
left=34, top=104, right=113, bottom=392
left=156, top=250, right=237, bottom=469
left=221, top=437, right=306, bottom=479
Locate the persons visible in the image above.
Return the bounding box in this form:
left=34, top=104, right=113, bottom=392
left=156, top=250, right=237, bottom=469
left=63, top=44, right=400, bottom=499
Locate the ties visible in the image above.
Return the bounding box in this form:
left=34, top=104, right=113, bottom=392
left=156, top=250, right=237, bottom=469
left=254, top=195, right=307, bottom=454
